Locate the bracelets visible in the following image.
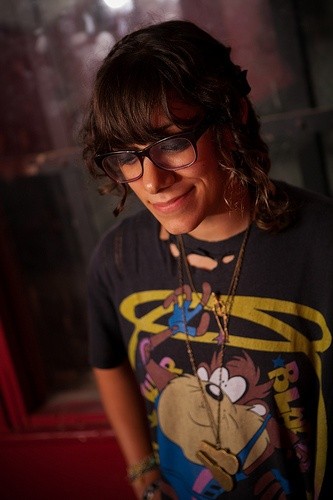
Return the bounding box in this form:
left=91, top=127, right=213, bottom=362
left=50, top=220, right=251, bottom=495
left=131, top=462, right=165, bottom=500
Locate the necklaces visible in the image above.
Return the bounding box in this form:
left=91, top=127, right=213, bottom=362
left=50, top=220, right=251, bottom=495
left=178, top=227, right=251, bottom=490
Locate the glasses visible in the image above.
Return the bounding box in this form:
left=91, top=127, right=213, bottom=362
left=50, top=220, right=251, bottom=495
left=93, top=109, right=222, bottom=184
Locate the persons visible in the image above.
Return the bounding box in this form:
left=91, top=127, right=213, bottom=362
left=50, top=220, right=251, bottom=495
left=81, top=20, right=333, bottom=500
